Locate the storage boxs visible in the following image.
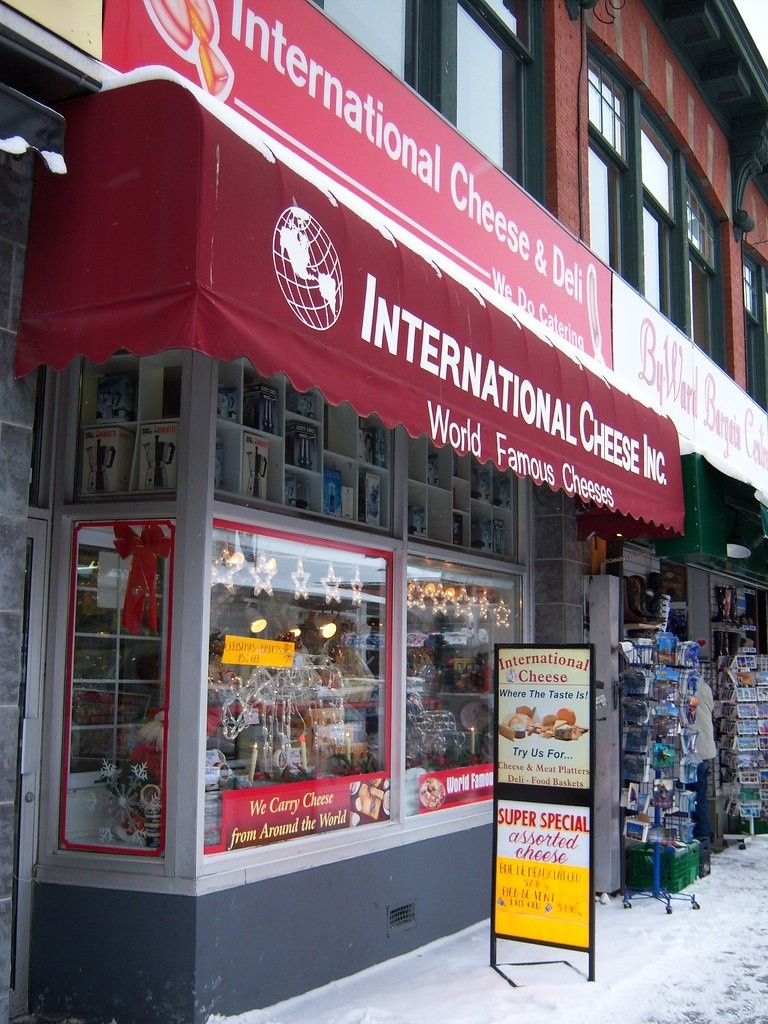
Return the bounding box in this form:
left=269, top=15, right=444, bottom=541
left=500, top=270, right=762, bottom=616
left=410, top=446, right=511, bottom=554
left=736, top=815, right=767, bottom=834
left=214, top=375, right=382, bottom=526
left=82, top=378, right=176, bottom=493
left=629, top=842, right=700, bottom=893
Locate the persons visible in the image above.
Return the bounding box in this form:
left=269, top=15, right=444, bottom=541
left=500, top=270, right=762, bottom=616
left=686, top=678, right=716, bottom=838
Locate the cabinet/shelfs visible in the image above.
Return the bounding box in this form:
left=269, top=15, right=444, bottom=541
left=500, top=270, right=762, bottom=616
left=77, top=346, right=516, bottom=559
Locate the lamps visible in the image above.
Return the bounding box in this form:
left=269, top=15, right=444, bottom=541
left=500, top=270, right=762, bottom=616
left=286, top=622, right=302, bottom=637
left=240, top=595, right=268, bottom=632
left=314, top=604, right=337, bottom=638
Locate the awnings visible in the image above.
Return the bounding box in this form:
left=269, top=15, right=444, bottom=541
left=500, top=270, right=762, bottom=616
left=14, top=78, right=686, bottom=538
